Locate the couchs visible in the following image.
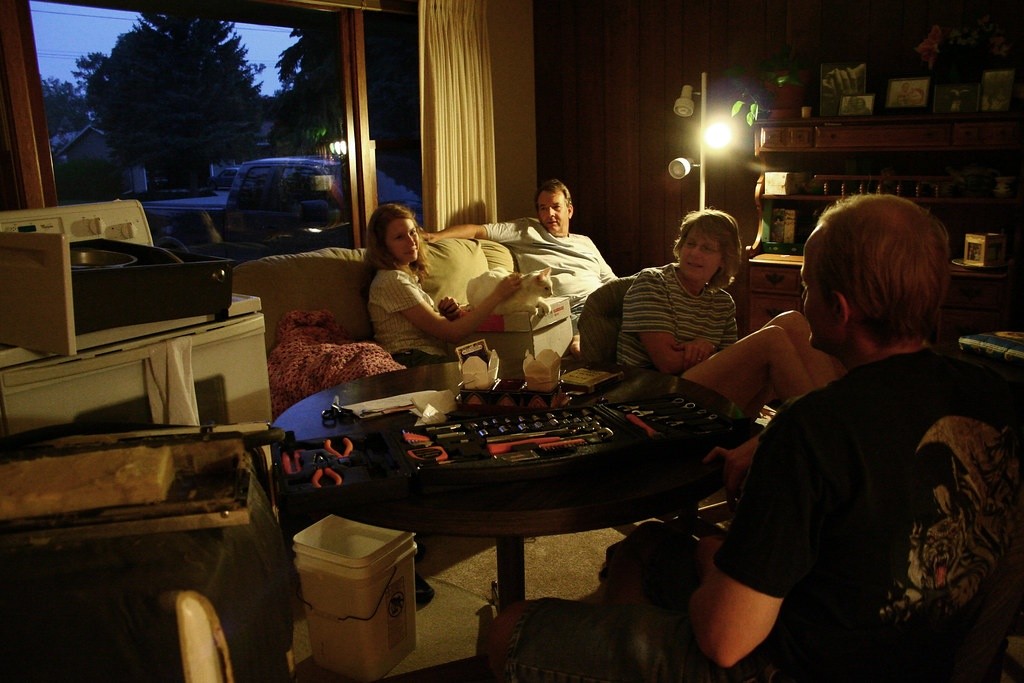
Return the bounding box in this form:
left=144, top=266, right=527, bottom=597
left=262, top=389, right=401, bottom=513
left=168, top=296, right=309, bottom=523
left=231, top=217, right=572, bottom=416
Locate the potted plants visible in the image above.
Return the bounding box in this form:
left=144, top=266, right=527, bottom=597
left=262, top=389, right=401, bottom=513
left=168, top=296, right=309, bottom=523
left=720, top=42, right=810, bottom=119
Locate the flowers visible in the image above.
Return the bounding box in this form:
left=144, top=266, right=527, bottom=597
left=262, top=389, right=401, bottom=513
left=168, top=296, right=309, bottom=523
left=913, top=14, right=1013, bottom=72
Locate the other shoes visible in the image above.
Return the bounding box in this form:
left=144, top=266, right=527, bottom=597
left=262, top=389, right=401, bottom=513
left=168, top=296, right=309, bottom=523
left=415, top=573, right=435, bottom=603
left=414, top=543, right=425, bottom=561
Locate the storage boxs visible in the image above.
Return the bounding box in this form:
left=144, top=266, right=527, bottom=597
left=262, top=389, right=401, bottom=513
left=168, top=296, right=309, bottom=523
left=71, top=238, right=235, bottom=336
left=457, top=298, right=569, bottom=331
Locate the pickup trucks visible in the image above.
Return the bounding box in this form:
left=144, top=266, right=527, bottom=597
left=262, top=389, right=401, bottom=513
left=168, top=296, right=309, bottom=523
left=145, top=155, right=422, bottom=245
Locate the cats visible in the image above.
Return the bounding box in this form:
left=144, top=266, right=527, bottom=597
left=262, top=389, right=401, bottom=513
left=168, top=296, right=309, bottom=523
left=467, top=267, right=553, bottom=314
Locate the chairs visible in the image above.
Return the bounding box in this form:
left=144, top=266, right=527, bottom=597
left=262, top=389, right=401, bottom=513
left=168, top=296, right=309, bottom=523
left=578, top=277, right=778, bottom=583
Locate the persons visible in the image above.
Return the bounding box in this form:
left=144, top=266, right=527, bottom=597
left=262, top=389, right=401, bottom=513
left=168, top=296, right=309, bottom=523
left=363, top=202, right=523, bottom=369
left=487, top=194, right=1024, bottom=683
left=414, top=180, right=617, bottom=360
left=616, top=209, right=840, bottom=408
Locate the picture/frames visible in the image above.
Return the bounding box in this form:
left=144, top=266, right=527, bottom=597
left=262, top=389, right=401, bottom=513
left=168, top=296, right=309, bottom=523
left=885, top=77, right=930, bottom=108
left=934, top=83, right=980, bottom=112
left=977, top=70, right=1015, bottom=112
left=819, top=60, right=867, bottom=117
left=838, top=93, right=875, bottom=116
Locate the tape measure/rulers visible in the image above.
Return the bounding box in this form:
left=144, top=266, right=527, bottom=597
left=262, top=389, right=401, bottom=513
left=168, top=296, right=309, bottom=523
left=407, top=444, right=449, bottom=465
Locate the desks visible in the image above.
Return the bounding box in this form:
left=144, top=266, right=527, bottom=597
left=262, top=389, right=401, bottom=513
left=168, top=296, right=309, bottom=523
left=270, top=360, right=746, bottom=608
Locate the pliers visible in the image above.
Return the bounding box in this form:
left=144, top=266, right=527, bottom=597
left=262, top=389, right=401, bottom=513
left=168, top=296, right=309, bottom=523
left=282, top=430, right=305, bottom=476
left=311, top=436, right=354, bottom=490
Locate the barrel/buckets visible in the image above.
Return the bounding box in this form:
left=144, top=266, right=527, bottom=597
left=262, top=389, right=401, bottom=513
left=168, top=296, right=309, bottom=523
left=292, top=513, right=417, bottom=683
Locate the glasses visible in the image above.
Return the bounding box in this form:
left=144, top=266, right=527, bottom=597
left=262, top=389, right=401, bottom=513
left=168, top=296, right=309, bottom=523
left=682, top=237, right=720, bottom=254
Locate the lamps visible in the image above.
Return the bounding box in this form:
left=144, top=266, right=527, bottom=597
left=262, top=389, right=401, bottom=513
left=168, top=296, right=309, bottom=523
left=669, top=71, right=707, bottom=211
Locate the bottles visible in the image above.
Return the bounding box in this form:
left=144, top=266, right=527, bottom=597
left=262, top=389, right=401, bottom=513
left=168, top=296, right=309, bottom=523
left=994, top=177, right=1017, bottom=196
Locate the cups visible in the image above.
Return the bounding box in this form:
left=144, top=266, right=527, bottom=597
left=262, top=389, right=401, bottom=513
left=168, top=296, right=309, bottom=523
left=801, top=106, right=812, bottom=118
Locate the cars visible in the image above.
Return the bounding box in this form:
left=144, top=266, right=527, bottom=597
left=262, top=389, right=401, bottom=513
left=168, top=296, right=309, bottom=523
left=207, top=167, right=239, bottom=189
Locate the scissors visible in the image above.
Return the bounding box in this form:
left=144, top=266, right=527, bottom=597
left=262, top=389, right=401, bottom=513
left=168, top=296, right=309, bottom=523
left=321, top=394, right=353, bottom=420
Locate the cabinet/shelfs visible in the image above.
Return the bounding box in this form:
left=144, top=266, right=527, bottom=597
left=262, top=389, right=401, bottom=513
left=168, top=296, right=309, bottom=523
left=743, top=113, right=1024, bottom=343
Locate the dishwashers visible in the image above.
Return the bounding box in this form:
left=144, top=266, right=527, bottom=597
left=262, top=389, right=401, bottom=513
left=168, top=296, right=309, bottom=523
left=0, top=199, right=274, bottom=437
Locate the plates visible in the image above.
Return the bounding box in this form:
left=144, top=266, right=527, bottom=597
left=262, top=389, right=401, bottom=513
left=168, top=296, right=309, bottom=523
left=951, top=258, right=1014, bottom=271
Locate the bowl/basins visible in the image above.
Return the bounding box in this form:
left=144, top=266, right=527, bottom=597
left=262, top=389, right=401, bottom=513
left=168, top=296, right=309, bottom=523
left=70, top=247, right=138, bottom=267
